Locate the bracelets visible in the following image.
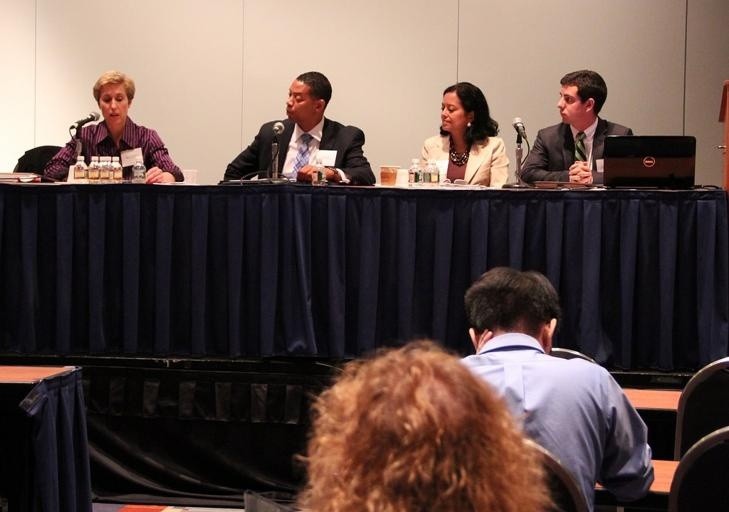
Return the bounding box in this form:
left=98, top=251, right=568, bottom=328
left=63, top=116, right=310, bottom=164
left=328, top=166, right=340, bottom=180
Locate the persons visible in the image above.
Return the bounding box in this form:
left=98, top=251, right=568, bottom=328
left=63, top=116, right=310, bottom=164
left=520, top=70, right=633, bottom=184
left=224, top=71, right=375, bottom=184
left=460, top=263, right=656, bottom=512
left=296, top=336, right=553, bottom=512
left=42, top=69, right=184, bottom=183
left=416, top=82, right=511, bottom=187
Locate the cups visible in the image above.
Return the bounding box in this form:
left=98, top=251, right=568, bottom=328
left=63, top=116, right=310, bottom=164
left=379, top=165, right=399, bottom=186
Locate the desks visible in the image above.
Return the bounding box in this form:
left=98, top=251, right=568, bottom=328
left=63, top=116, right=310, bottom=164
left=1, top=172, right=727, bottom=374
left=597, top=456, right=676, bottom=507
left=0, top=363, right=98, bottom=511
left=618, top=388, right=686, bottom=415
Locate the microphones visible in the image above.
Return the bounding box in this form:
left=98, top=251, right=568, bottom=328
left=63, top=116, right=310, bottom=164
left=512, top=117, right=527, bottom=138
left=70, top=112, right=100, bottom=129
left=272, top=122, right=285, bottom=136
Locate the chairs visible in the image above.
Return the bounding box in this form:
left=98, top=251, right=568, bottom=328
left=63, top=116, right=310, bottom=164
left=12, top=145, right=65, bottom=174
left=669, top=425, right=729, bottom=508
left=518, top=434, right=589, bottom=510
left=675, top=357, right=728, bottom=459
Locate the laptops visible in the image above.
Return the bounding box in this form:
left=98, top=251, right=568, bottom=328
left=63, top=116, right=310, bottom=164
left=603, top=136, right=696, bottom=189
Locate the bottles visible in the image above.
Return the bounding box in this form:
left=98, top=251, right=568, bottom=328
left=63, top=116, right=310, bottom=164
left=72, top=155, right=125, bottom=184
left=310, top=157, right=327, bottom=187
left=131, top=154, right=148, bottom=184
left=407, top=158, right=440, bottom=183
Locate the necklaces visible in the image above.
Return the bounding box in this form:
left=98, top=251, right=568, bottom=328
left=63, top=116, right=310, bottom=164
left=449, top=140, right=469, bottom=166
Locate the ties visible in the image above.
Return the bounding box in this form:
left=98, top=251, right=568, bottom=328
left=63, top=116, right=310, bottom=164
left=574, top=132, right=587, bottom=162
left=292, top=133, right=313, bottom=181
